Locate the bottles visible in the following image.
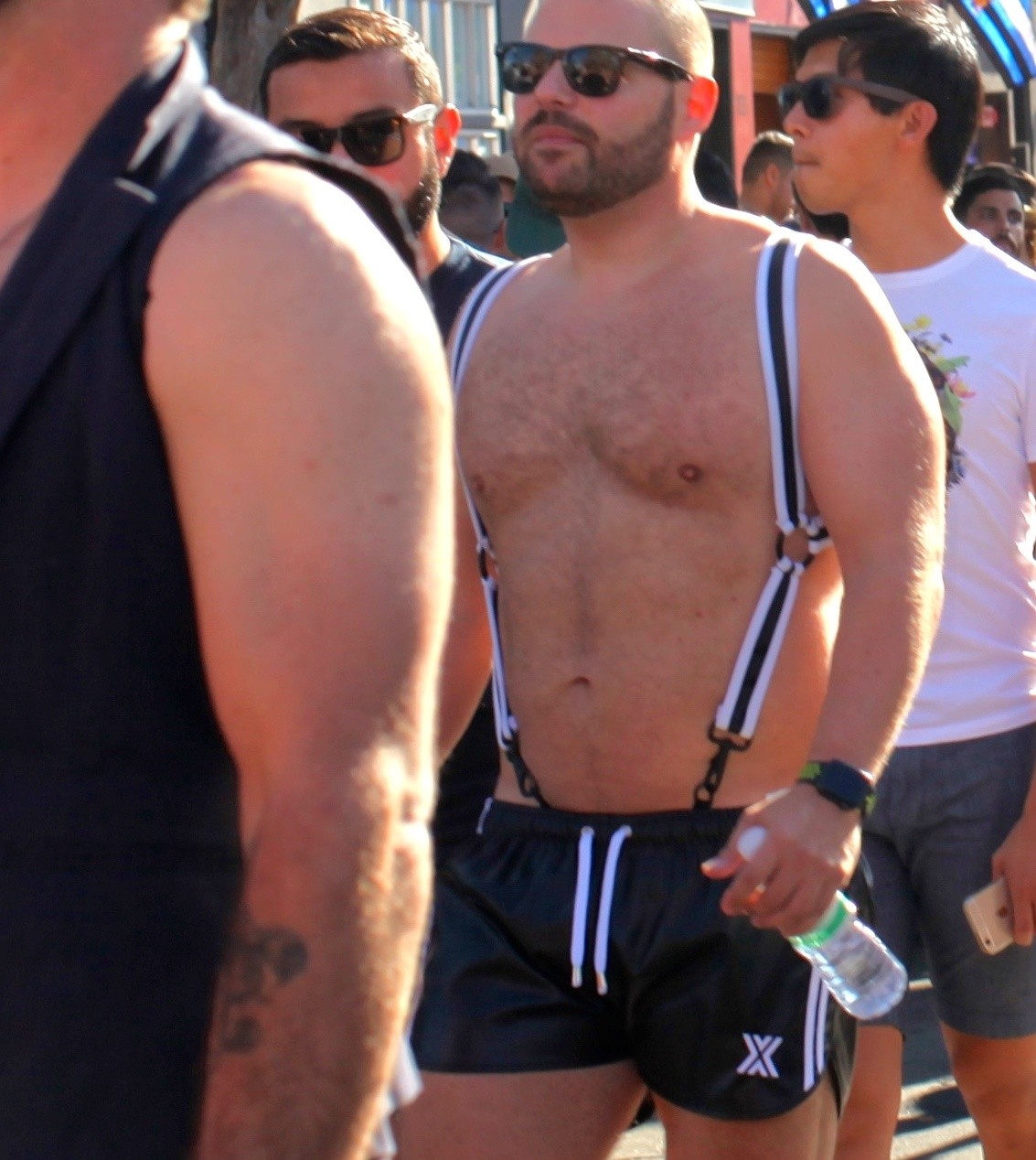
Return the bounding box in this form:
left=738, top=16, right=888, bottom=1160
left=735, top=826, right=908, bottom=1019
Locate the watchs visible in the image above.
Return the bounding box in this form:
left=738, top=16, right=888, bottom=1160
left=797, top=758, right=878, bottom=821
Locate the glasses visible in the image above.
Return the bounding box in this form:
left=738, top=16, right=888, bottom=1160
left=495, top=41, right=697, bottom=97
left=776, top=72, right=920, bottom=121
left=277, top=104, right=440, bottom=167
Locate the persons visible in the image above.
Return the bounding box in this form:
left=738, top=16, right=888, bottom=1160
left=0, top=0, right=453, bottom=1160
left=696, top=131, right=848, bottom=243
left=262, top=8, right=565, bottom=851
left=953, top=161, right=1036, bottom=270
left=782, top=0, right=1036, bottom=1160
left=386, top=0, right=942, bottom=1160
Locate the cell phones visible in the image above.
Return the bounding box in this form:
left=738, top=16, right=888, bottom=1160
left=963, top=876, right=1016, bottom=954
